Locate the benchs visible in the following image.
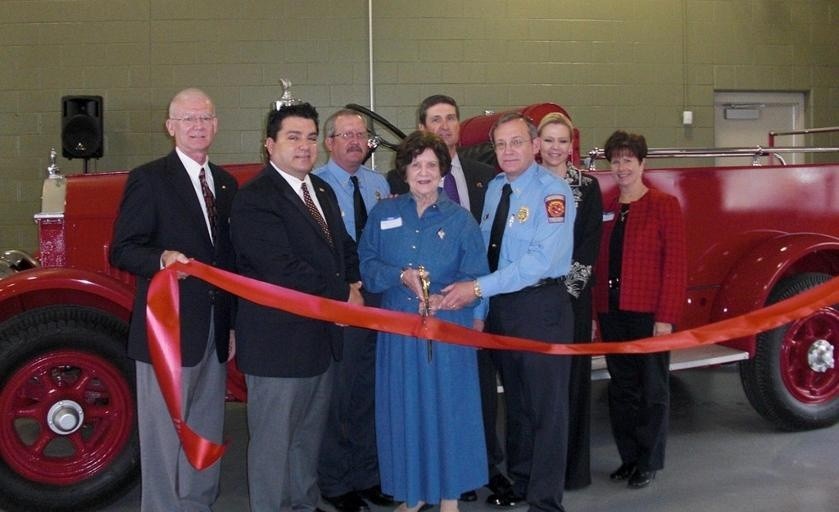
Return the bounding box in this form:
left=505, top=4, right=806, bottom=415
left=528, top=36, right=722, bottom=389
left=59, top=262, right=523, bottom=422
left=453, top=103, right=579, bottom=176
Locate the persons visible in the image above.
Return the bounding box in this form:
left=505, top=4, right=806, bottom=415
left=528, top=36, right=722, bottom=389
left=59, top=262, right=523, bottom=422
left=591, top=129, right=687, bottom=489
left=232, top=102, right=361, bottom=512
left=385, top=94, right=512, bottom=501
left=438, top=113, right=578, bottom=510
left=309, top=109, right=394, bottom=512
left=530, top=112, right=604, bottom=491
left=106, top=89, right=242, bottom=511
left=356, top=131, right=491, bottom=512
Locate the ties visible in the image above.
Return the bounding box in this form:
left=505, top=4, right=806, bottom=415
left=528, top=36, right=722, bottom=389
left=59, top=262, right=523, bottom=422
left=488, top=184, right=513, bottom=273
left=199, top=169, right=217, bottom=247
left=350, top=176, right=368, bottom=244
left=443, top=166, right=461, bottom=204
left=301, top=183, right=339, bottom=255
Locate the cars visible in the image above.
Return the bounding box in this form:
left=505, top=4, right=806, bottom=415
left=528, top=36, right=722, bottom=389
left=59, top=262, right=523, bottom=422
left=0, top=101, right=839, bottom=506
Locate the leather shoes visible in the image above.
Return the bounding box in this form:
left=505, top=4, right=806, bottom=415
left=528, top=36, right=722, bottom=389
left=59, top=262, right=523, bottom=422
left=320, top=492, right=371, bottom=512
left=458, top=490, right=478, bottom=502
left=610, top=463, right=635, bottom=481
left=356, top=485, right=395, bottom=506
left=627, top=468, right=656, bottom=489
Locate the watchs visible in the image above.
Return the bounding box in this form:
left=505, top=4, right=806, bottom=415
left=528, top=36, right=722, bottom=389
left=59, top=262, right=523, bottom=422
left=473, top=280, right=484, bottom=299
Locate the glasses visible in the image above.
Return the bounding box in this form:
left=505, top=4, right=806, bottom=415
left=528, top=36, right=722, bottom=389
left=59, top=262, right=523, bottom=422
left=408, top=160, right=440, bottom=169
left=329, top=131, right=367, bottom=139
left=170, top=114, right=218, bottom=125
left=493, top=138, right=533, bottom=150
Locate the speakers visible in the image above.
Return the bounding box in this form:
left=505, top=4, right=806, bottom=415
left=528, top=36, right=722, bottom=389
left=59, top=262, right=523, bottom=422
left=61, top=95, right=104, bottom=161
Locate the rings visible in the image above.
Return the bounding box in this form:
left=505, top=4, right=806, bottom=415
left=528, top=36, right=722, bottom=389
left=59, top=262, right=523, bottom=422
left=180, top=275, right=186, bottom=279
left=452, top=306, right=456, bottom=310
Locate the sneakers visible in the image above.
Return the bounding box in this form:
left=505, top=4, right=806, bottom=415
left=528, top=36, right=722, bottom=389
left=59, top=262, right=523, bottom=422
left=485, top=487, right=529, bottom=508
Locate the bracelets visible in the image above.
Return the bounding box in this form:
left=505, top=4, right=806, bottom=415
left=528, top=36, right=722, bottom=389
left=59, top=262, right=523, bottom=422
left=399, top=270, right=406, bottom=285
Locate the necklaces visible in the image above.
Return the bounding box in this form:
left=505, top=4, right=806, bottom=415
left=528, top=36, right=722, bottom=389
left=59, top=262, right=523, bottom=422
left=618, top=207, right=630, bottom=222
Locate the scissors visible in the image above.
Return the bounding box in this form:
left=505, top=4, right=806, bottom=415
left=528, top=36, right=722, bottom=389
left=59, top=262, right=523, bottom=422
left=418, top=264, right=431, bottom=325
